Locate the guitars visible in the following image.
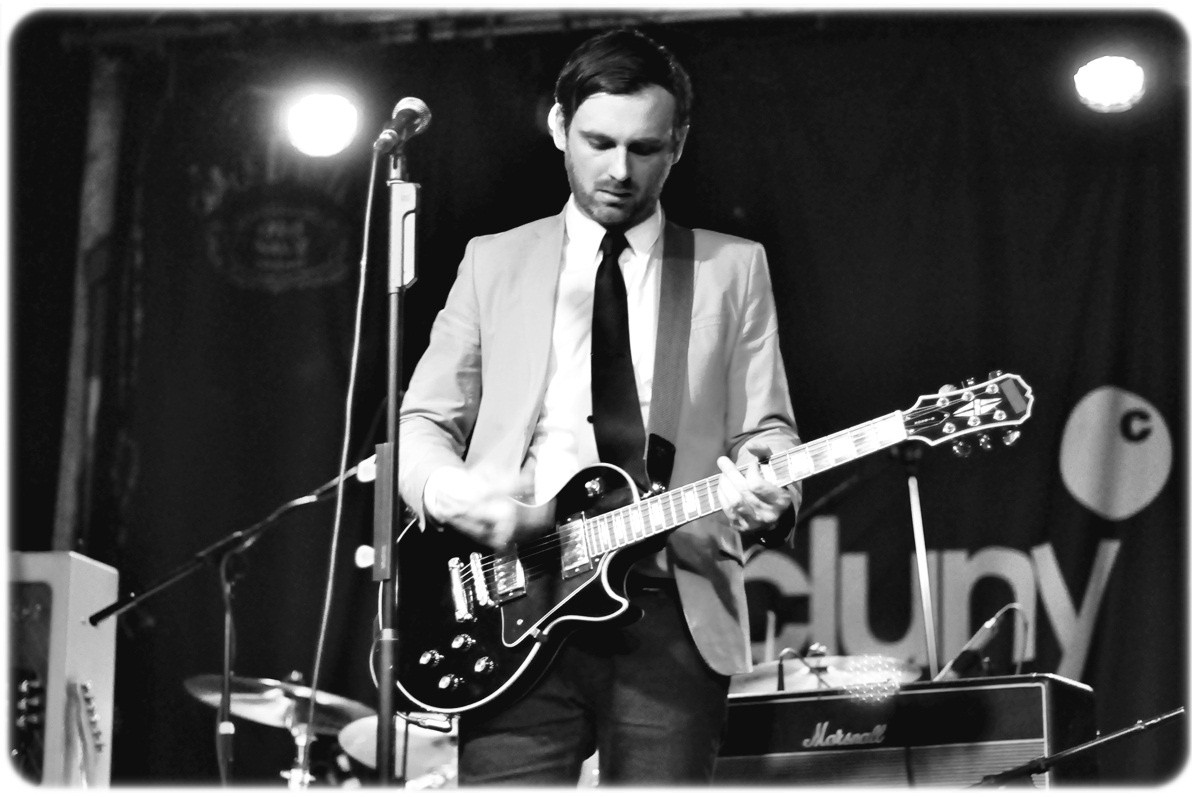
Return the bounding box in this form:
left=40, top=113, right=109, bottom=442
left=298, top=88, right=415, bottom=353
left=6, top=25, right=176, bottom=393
left=374, top=368, right=1037, bottom=715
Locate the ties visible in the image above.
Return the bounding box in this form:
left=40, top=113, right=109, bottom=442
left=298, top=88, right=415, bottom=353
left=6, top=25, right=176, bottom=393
left=591, top=233, right=650, bottom=497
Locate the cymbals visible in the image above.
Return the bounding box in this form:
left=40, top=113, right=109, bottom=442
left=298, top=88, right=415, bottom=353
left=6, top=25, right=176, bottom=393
left=724, top=652, right=922, bottom=698
left=337, top=712, right=602, bottom=790
left=184, top=672, right=373, bottom=736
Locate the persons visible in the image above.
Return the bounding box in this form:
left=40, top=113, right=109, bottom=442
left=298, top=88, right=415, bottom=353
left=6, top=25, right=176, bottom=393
left=395, top=29, right=805, bottom=786
